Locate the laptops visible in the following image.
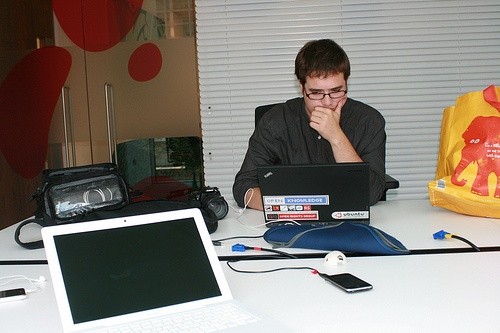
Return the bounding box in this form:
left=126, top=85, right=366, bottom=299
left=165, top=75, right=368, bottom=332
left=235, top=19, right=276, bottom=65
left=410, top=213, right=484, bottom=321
left=256, top=161, right=370, bottom=227
left=41, top=208, right=264, bottom=333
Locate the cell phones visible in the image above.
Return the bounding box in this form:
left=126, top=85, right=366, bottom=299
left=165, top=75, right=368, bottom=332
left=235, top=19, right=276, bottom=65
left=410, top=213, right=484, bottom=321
left=325, top=273, right=373, bottom=293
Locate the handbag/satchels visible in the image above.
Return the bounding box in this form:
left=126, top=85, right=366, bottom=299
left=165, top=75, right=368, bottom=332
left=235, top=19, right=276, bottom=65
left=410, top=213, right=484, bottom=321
left=33, top=162, right=130, bottom=225
left=428, top=85, right=500, bottom=219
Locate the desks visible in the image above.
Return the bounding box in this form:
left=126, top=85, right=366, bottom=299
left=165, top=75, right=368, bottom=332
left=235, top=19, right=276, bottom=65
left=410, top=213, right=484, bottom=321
left=0, top=199, right=500, bottom=333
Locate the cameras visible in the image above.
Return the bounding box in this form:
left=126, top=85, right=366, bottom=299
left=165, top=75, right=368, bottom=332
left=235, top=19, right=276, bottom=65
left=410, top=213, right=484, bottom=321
left=187, top=186, right=229, bottom=220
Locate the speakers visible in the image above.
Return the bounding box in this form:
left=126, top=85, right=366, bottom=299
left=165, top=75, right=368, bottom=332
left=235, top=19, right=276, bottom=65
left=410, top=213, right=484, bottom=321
left=112, top=136, right=205, bottom=188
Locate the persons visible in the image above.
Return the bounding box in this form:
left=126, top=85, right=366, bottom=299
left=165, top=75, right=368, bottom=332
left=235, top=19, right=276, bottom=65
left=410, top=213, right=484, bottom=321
left=233, top=39, right=386, bottom=210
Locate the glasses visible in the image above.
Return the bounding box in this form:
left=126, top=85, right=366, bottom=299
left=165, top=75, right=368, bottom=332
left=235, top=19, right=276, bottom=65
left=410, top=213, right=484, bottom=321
left=302, top=83, right=348, bottom=101
